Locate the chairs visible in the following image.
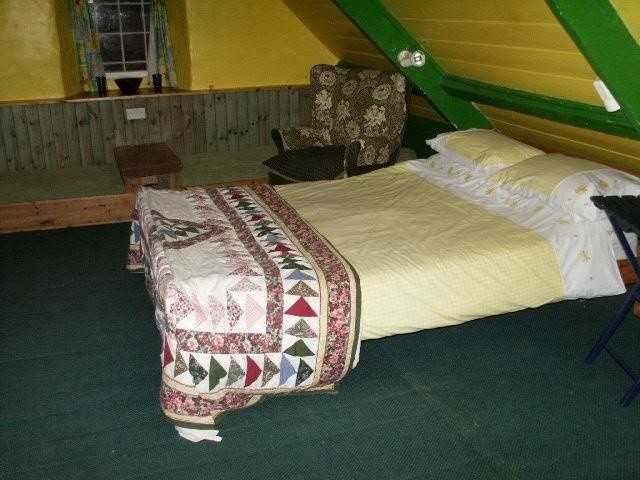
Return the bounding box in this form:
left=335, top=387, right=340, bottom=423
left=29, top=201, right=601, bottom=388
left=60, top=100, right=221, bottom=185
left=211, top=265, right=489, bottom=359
left=262, top=64, right=411, bottom=184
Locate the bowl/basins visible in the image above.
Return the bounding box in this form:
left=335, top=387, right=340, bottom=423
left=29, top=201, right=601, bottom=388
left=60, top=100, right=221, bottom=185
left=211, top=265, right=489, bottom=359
left=114, top=77, right=142, bottom=93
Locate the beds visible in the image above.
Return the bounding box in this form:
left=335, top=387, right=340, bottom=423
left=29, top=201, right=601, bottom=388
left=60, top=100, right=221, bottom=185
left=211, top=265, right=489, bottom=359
left=125, top=153, right=639, bottom=429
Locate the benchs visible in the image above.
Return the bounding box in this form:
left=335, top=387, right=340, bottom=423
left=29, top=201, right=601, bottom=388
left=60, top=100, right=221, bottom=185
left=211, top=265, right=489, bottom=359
left=0, top=84, right=310, bottom=233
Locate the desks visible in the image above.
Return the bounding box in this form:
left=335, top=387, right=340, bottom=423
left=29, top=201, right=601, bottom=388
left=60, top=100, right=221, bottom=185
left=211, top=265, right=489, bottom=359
left=113, top=142, right=183, bottom=194
left=583, top=194, right=640, bottom=407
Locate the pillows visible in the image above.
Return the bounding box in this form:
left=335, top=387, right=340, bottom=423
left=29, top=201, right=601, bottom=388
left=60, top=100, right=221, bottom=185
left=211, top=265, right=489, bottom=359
left=489, top=152, right=640, bottom=221
left=424, top=127, right=545, bottom=176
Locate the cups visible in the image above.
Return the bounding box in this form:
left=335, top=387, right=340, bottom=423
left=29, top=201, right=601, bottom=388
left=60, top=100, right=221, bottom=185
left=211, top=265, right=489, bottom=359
left=152, top=74, right=162, bottom=91
left=95, top=76, right=106, bottom=95
